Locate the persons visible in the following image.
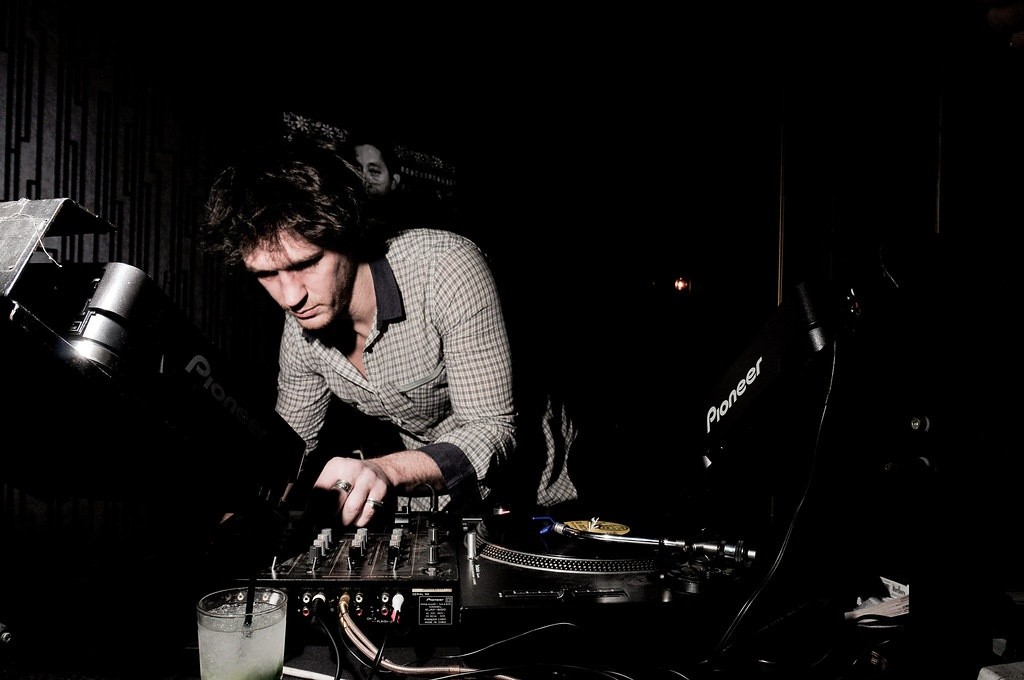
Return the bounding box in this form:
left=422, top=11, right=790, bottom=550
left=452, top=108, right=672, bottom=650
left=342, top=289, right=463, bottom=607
left=202, top=163, right=579, bottom=609
left=348, top=141, right=399, bottom=205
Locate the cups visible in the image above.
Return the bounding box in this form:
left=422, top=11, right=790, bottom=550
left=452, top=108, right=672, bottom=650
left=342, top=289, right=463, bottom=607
left=196, top=586, right=288, bottom=680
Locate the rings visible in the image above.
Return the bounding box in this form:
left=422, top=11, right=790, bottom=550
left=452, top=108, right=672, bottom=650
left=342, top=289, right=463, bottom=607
left=341, top=481, right=353, bottom=493
